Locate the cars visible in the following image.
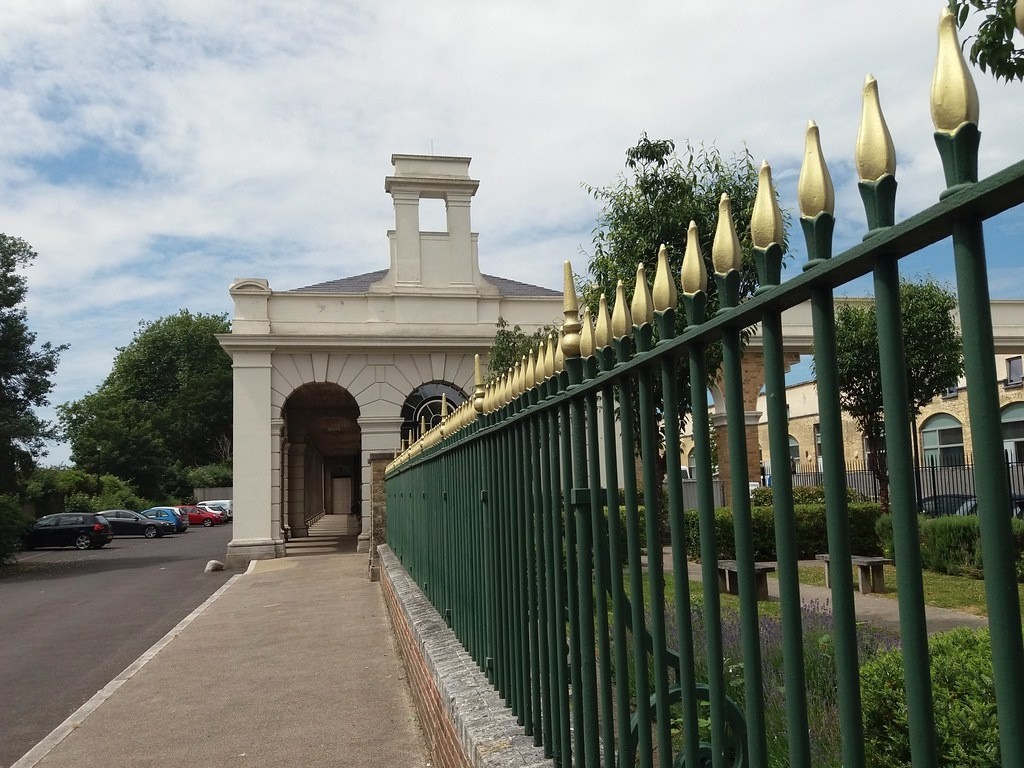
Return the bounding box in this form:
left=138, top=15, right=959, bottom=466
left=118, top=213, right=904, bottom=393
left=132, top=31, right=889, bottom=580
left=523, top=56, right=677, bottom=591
left=95, top=509, right=176, bottom=538
left=953, top=495, right=1024, bottom=522
left=15, top=512, right=113, bottom=550
left=196, top=505, right=228, bottom=522
left=174, top=505, right=222, bottom=527
left=130, top=508, right=184, bottom=535
left=145, top=507, right=190, bottom=532
left=916, top=494, right=971, bottom=518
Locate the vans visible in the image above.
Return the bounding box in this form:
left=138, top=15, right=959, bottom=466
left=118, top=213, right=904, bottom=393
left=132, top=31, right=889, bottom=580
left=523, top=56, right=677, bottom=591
left=196, top=500, right=233, bottom=519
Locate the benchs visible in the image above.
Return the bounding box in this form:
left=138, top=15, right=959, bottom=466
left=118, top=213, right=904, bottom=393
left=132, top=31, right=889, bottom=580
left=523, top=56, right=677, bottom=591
left=717, top=560, right=775, bottom=601
left=815, top=554, right=894, bottom=594
